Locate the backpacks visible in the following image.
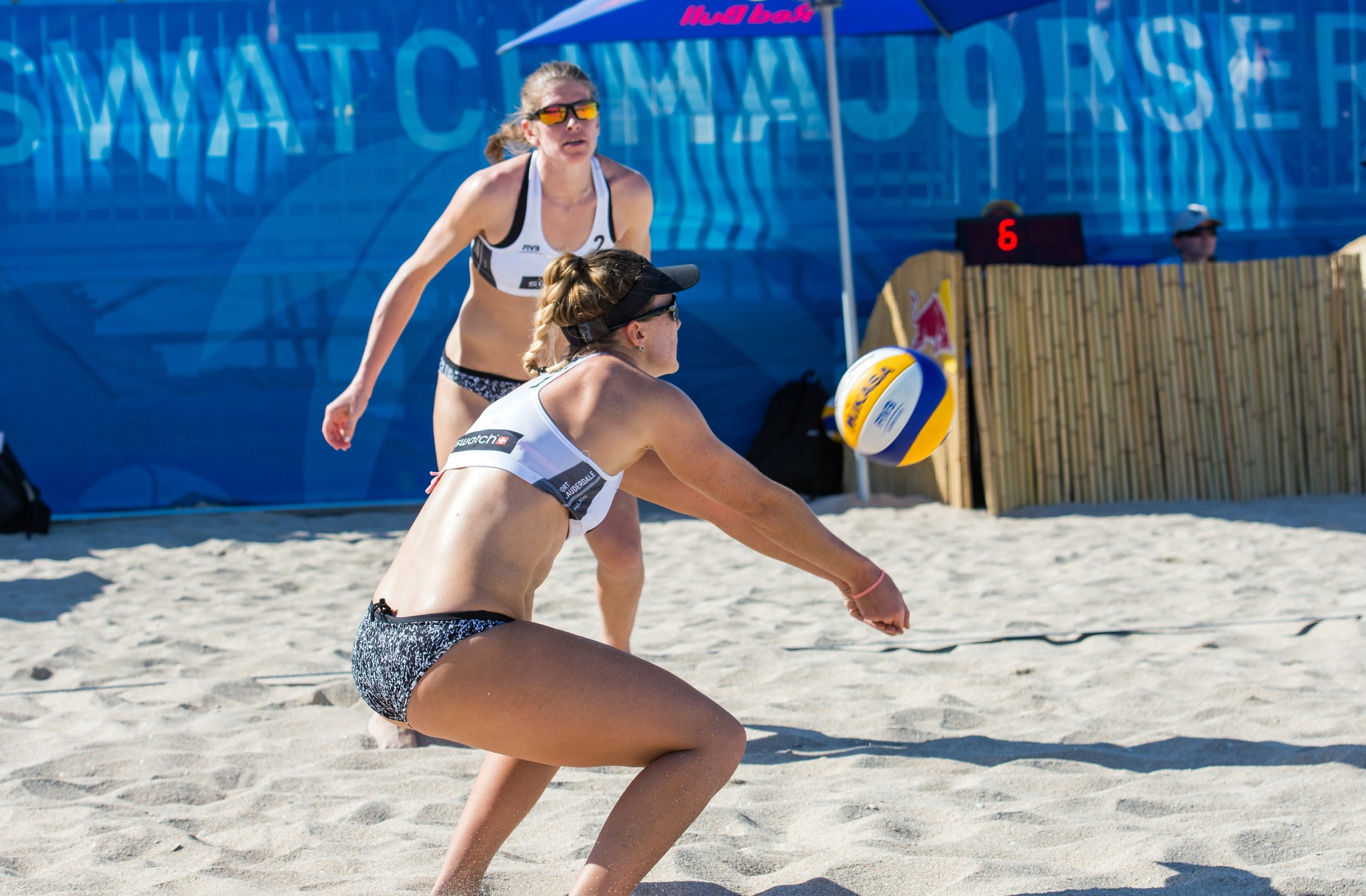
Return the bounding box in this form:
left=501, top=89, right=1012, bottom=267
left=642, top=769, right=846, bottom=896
left=0, top=433, right=50, bottom=537
left=745, top=370, right=844, bottom=499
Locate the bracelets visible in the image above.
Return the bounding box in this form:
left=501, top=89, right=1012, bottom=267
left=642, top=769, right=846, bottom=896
left=851, top=569, right=884, bottom=598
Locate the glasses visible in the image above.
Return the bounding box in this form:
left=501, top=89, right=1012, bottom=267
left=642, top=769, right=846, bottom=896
left=609, top=293, right=678, bottom=329
left=528, top=99, right=599, bottom=126
left=1179, top=226, right=1216, bottom=237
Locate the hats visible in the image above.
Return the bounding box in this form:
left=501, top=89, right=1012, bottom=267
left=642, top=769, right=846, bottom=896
left=1175, top=203, right=1222, bottom=232
left=561, top=255, right=700, bottom=345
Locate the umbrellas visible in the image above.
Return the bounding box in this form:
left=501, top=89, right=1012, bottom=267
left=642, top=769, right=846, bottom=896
left=497, top=0, right=1052, bottom=505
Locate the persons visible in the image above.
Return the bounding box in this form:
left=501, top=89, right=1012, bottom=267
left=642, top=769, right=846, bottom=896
left=1159, top=202, right=1222, bottom=264
left=320, top=62, right=652, bottom=750
left=352, top=249, right=908, bottom=896
left=980, top=200, right=1023, bottom=215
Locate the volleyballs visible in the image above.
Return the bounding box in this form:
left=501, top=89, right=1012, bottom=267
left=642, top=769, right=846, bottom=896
left=832, top=345, right=956, bottom=469
